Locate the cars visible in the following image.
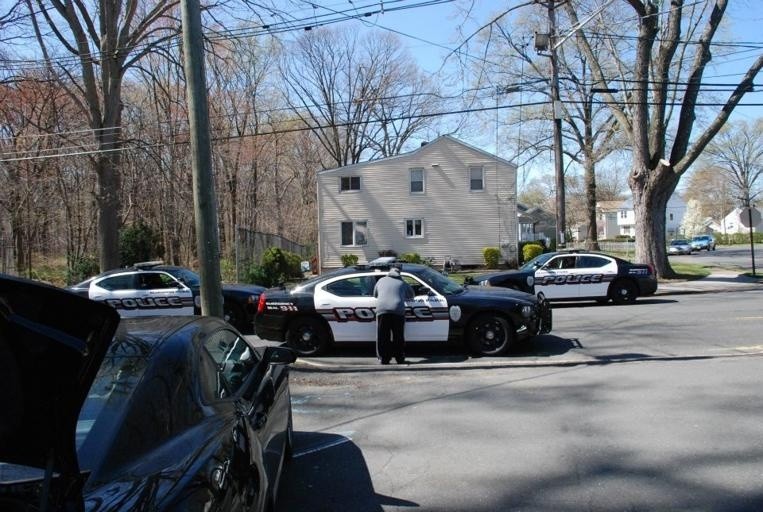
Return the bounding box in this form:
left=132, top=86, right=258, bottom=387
left=667, top=239, right=692, bottom=255
left=0, top=273, right=297, bottom=512
left=63, top=260, right=269, bottom=335
left=254, top=256, right=552, bottom=357
left=464, top=248, right=658, bottom=305
left=689, top=235, right=716, bottom=251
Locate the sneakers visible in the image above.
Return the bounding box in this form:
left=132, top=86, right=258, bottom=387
left=398, top=360, right=404, bottom=364
left=380, top=360, right=389, bottom=364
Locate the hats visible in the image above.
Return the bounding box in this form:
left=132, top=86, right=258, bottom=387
left=388, top=268, right=400, bottom=279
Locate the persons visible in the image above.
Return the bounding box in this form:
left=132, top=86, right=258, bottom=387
left=373, top=267, right=415, bottom=364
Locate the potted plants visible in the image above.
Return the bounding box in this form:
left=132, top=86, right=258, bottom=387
left=484, top=247, right=500, bottom=269
